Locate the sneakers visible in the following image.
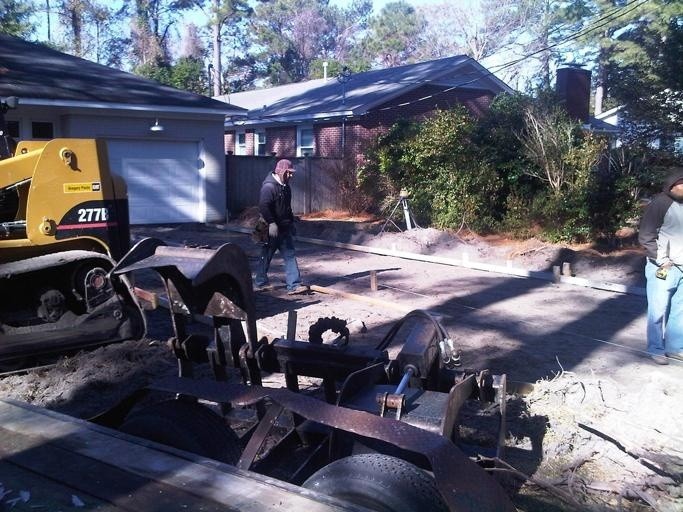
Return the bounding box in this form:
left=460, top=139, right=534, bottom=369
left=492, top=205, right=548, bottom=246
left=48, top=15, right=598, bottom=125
left=667, top=349, right=682, bottom=361
left=651, top=354, right=667, bottom=364
left=288, top=286, right=309, bottom=294
left=260, top=283, right=274, bottom=292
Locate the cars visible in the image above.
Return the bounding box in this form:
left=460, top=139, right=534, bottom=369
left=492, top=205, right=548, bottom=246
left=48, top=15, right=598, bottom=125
left=581, top=87, right=683, bottom=155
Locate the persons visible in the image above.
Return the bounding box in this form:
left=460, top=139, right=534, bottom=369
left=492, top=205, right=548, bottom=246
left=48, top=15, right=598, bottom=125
left=639, top=170, right=683, bottom=365
left=255, top=159, right=308, bottom=294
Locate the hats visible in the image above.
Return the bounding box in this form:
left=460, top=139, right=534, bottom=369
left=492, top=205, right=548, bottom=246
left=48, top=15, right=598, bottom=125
left=274, top=160, right=297, bottom=176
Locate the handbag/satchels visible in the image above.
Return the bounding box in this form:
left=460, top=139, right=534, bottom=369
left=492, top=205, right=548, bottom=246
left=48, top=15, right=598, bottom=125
left=252, top=217, right=270, bottom=243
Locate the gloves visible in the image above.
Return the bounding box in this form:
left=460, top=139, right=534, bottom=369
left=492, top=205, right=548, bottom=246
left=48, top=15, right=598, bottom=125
left=269, top=223, right=278, bottom=239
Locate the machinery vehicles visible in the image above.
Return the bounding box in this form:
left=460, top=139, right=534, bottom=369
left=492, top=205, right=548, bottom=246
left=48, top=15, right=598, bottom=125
left=0, top=129, right=148, bottom=378
left=0, top=238, right=517, bottom=511
